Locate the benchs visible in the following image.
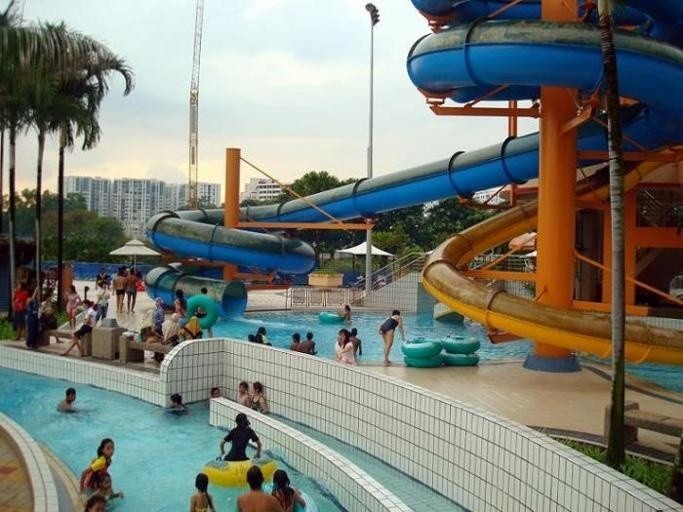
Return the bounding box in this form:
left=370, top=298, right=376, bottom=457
left=37, top=328, right=91, bottom=356
left=601, top=399, right=683, bottom=462
left=119, top=332, right=176, bottom=366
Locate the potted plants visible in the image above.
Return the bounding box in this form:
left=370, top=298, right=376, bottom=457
left=308, top=259, right=344, bottom=289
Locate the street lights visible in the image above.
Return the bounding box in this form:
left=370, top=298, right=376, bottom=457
left=363, top=3, right=380, bottom=298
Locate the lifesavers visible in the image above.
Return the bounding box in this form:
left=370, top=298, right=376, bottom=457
left=260, top=483, right=320, bottom=512
left=204, top=458, right=277, bottom=487
left=319, top=311, right=344, bottom=323
left=401, top=335, right=481, bottom=366
left=186, top=295, right=217, bottom=329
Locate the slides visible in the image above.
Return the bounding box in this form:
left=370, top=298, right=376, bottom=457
left=144, top=1, right=681, bottom=365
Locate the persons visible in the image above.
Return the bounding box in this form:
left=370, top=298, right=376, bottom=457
left=291, top=332, right=318, bottom=355
left=171, top=394, right=184, bottom=408
left=79, top=438, right=124, bottom=512
left=379, top=310, right=405, bottom=363
left=219, top=413, right=261, bottom=461
left=236, top=381, right=268, bottom=413
left=210, top=388, right=220, bottom=398
left=236, top=466, right=306, bottom=512
left=248, top=327, right=272, bottom=346
left=13, top=264, right=213, bottom=361
left=341, top=304, right=352, bottom=321
left=335, top=327, right=363, bottom=364
left=190, top=473, right=215, bottom=512
left=59, top=387, right=76, bottom=409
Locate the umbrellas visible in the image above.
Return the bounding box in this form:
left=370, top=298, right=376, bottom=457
left=108, top=237, right=162, bottom=274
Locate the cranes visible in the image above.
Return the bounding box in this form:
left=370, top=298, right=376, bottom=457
left=185, top=0, right=206, bottom=210
